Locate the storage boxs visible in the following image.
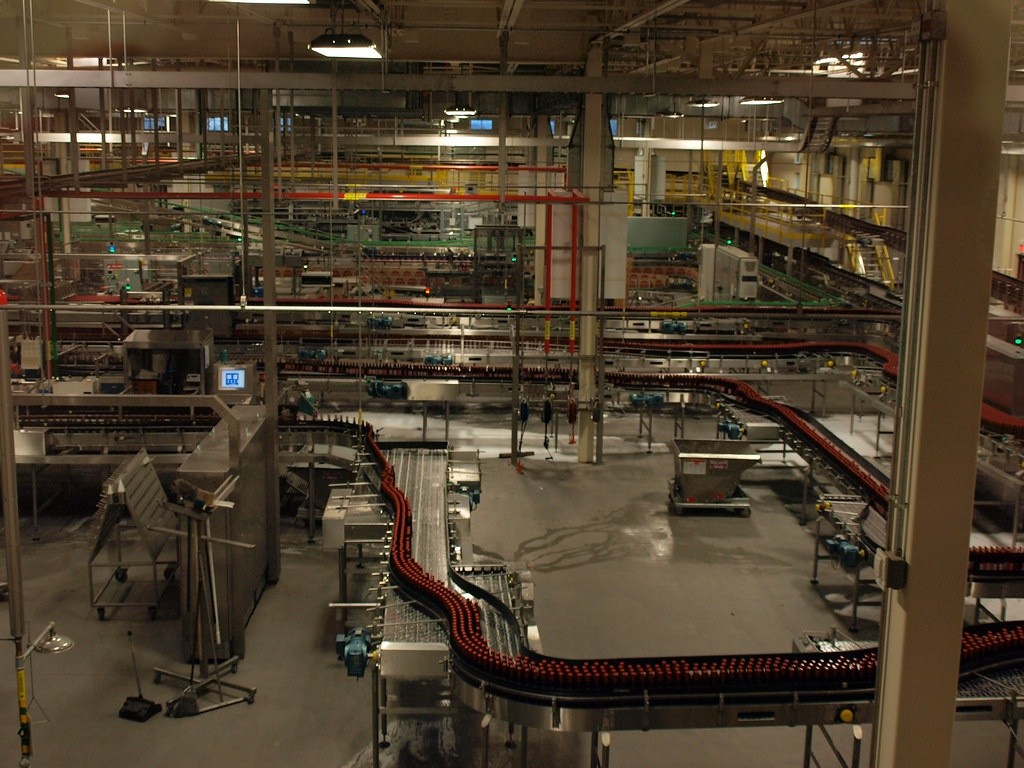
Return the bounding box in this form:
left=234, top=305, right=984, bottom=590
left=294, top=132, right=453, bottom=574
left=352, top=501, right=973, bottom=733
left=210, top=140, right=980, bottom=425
left=251, top=265, right=699, bottom=291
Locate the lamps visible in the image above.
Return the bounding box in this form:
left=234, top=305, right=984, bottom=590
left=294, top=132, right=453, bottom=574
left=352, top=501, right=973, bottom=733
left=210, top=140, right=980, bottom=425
left=443, top=92, right=477, bottom=116
left=655, top=97, right=684, bottom=119
left=306, top=0, right=385, bottom=60
left=685, top=99, right=720, bottom=108
left=738, top=96, right=784, bottom=105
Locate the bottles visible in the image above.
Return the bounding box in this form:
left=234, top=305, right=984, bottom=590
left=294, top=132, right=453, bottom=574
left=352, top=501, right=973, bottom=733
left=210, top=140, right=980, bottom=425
left=981, top=404, right=1024, bottom=439
left=276, top=298, right=580, bottom=314
left=502, top=653, right=879, bottom=699
left=960, top=626, right=1024, bottom=674
left=967, top=545, right=1024, bottom=576
left=666, top=165, right=908, bottom=261
left=733, top=377, right=890, bottom=522
left=992, top=272, right=1024, bottom=304
left=17, top=414, right=221, bottom=434
left=843, top=309, right=899, bottom=383
left=281, top=417, right=501, bottom=700
left=473, top=366, right=732, bottom=397
left=229, top=357, right=470, bottom=386
left=605, top=304, right=842, bottom=317
left=603, top=339, right=844, bottom=360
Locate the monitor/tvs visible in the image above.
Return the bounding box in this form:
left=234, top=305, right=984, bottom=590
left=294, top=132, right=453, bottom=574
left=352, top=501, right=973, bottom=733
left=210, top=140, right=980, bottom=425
left=217, top=367, right=247, bottom=390
